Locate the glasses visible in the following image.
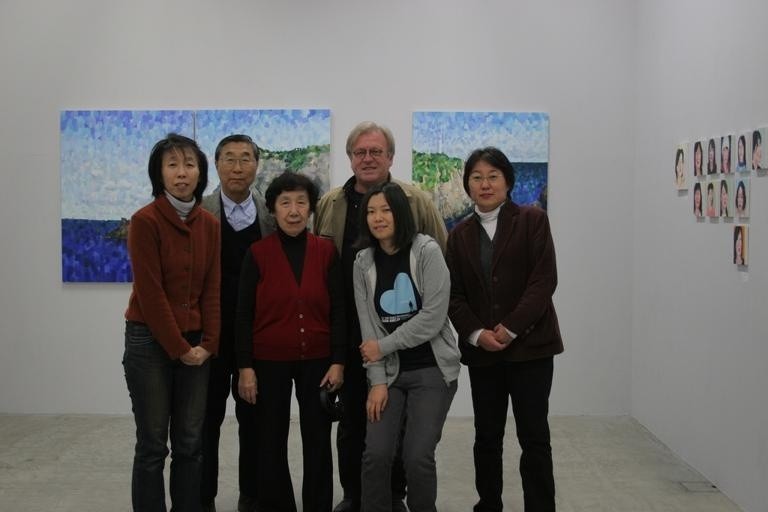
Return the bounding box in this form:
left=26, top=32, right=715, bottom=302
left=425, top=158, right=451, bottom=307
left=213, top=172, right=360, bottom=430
left=469, top=171, right=504, bottom=183
left=218, top=157, right=257, bottom=168
left=350, top=147, right=391, bottom=158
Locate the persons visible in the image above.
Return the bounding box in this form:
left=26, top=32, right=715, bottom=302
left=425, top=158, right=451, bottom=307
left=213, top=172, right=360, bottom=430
left=191, top=133, right=282, bottom=511
left=441, top=146, right=564, bottom=512
left=689, top=130, right=763, bottom=218
left=732, top=226, right=744, bottom=265
left=305, top=119, right=451, bottom=512
left=232, top=167, right=347, bottom=510
left=674, top=146, right=687, bottom=188
left=349, top=180, right=464, bottom=512
left=121, top=132, right=222, bottom=512
